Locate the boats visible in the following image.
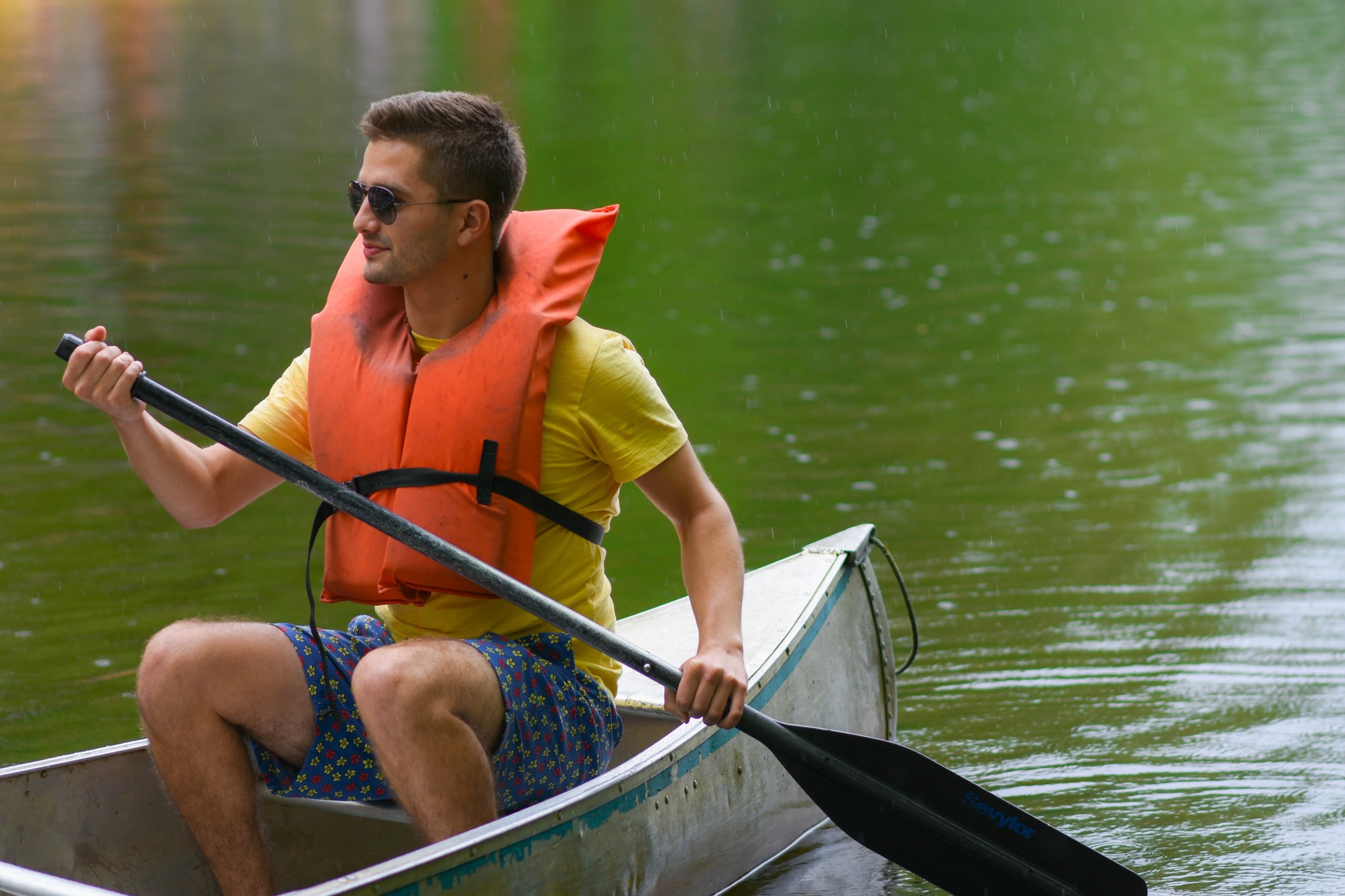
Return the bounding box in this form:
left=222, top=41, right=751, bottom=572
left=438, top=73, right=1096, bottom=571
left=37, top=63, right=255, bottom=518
left=0, top=515, right=921, bottom=896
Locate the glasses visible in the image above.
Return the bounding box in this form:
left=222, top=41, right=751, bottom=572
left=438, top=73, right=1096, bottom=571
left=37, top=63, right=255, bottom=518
left=347, top=180, right=494, bottom=225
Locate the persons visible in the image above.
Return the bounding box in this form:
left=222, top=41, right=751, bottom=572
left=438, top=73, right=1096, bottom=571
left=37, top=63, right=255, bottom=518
left=61, top=92, right=748, bottom=896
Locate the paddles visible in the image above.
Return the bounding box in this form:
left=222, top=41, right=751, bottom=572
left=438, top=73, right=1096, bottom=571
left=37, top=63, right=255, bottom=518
left=52, top=327, right=1153, bottom=896
